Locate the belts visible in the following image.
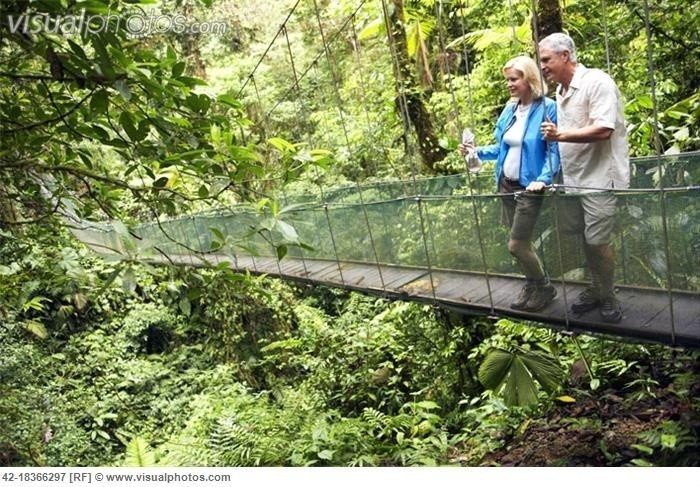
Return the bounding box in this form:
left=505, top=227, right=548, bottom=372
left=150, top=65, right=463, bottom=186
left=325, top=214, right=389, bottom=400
left=502, top=171, right=519, bottom=186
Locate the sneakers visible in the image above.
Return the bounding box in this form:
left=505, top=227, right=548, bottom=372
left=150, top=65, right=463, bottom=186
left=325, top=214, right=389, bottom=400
left=571, top=288, right=603, bottom=313
left=524, top=284, right=558, bottom=312
left=508, top=279, right=538, bottom=310
left=599, top=296, right=622, bottom=324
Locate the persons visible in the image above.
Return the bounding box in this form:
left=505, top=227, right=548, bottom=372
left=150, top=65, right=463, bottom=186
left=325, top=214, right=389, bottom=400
left=459, top=32, right=632, bottom=324
